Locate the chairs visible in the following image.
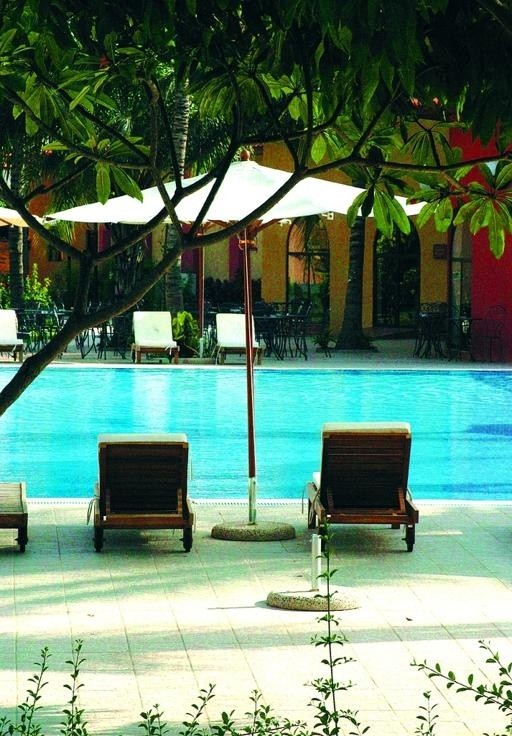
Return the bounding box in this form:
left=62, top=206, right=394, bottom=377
left=412, top=302, right=507, bottom=362
left=93, top=433, right=196, bottom=552
left=306, top=422, right=418, bottom=553
left=0, top=297, right=313, bottom=364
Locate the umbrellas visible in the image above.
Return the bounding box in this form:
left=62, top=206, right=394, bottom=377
left=49, top=147, right=440, bottom=543
left=0, top=207, right=49, bottom=228
